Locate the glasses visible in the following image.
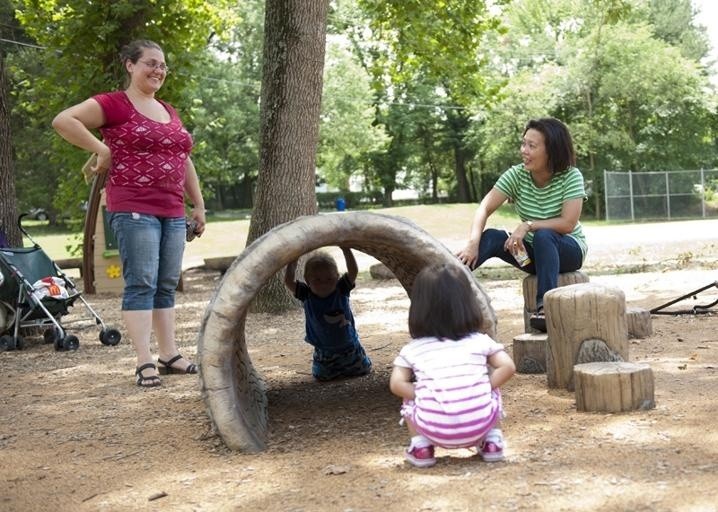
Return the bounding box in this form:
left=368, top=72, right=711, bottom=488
left=138, top=59, right=169, bottom=71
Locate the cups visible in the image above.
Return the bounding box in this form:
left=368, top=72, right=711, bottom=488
left=508, top=241, right=532, bottom=268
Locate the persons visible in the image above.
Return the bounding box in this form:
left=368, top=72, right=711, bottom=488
left=51, top=38, right=207, bottom=387
left=389, top=263, right=517, bottom=468
left=451, top=117, right=591, bottom=334
left=283, top=244, right=373, bottom=382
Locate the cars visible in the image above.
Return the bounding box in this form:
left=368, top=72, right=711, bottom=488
left=25, top=203, right=88, bottom=220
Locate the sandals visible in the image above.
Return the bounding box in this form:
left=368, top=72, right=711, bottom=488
left=157, top=354, right=198, bottom=374
left=134, top=360, right=161, bottom=386
left=529, top=306, right=547, bottom=333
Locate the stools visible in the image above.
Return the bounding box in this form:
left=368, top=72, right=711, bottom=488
left=523, top=269, right=589, bottom=333
left=546, top=281, right=628, bottom=390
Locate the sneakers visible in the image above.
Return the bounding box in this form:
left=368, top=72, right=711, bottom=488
left=475, top=434, right=506, bottom=463
left=402, top=442, right=437, bottom=469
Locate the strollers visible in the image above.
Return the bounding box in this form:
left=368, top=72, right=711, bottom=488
left=0, top=212, right=121, bottom=351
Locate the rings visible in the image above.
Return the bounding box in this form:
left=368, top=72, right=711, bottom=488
left=513, top=242, right=517, bottom=247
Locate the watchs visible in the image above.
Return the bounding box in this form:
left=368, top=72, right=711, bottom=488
left=524, top=220, right=534, bottom=232
